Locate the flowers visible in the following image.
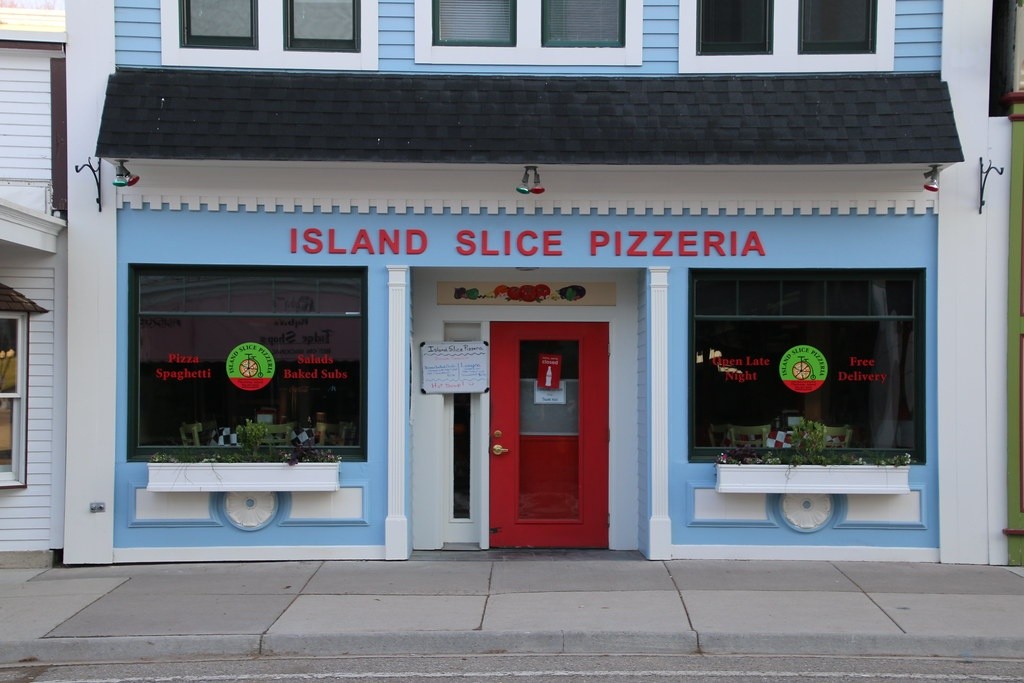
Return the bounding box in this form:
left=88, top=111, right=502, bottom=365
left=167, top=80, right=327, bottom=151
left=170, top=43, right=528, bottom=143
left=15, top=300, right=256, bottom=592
left=716, top=416, right=911, bottom=465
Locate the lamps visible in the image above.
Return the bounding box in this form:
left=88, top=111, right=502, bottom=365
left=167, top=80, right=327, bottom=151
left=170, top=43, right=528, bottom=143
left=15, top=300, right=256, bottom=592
left=515, top=165, right=545, bottom=195
left=923, top=165, right=939, bottom=191
left=112, top=159, right=139, bottom=186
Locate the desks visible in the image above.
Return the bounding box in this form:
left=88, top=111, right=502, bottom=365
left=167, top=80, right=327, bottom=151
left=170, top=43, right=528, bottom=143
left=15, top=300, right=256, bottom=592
left=207, top=426, right=315, bottom=445
left=721, top=430, right=846, bottom=448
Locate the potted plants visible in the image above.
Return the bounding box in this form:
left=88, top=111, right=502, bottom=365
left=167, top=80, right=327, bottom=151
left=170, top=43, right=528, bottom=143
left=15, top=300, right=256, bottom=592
left=714, top=463, right=909, bottom=494
left=146, top=419, right=341, bottom=492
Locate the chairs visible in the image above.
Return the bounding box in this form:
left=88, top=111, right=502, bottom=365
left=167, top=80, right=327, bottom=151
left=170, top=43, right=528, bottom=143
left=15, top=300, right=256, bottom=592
left=178, top=423, right=202, bottom=446
left=710, top=425, right=772, bottom=447
left=823, top=426, right=853, bottom=448
left=261, top=423, right=295, bottom=445
